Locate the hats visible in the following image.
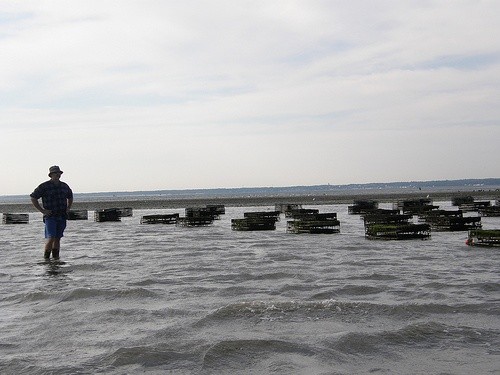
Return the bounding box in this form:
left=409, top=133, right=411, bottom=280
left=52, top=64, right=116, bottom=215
left=48, top=166, right=63, bottom=176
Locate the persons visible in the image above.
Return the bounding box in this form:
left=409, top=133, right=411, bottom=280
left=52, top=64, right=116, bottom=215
left=30, top=165, right=73, bottom=259
